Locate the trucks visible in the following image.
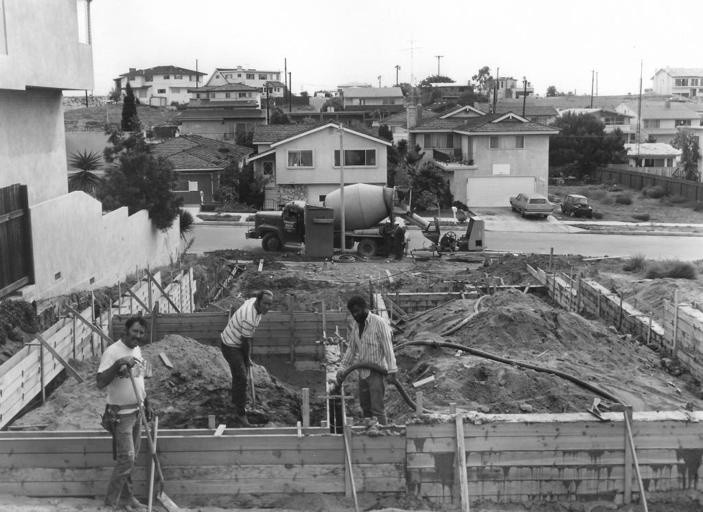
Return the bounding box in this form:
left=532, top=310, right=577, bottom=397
left=244, top=182, right=431, bottom=258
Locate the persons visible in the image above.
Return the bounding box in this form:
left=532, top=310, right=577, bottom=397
left=220, top=289, right=273, bottom=416
left=94, top=317, right=153, bottom=511
left=335, top=295, right=398, bottom=434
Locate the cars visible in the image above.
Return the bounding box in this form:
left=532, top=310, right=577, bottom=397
left=562, top=194, right=593, bottom=218
left=509, top=192, right=554, bottom=218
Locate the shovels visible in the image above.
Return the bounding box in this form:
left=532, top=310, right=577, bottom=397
left=128, top=365, right=180, bottom=512
left=244, top=365, right=268, bottom=424
